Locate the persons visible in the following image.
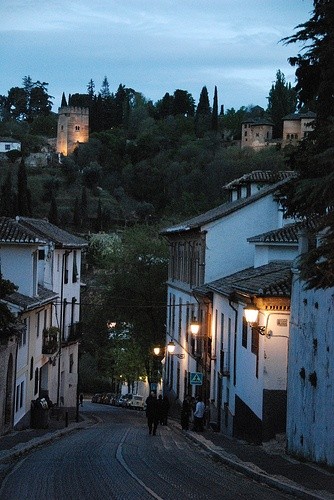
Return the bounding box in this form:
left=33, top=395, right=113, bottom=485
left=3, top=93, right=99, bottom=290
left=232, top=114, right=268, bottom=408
left=145, top=391, right=171, bottom=436
left=79, top=393, right=84, bottom=407
left=181, top=394, right=206, bottom=433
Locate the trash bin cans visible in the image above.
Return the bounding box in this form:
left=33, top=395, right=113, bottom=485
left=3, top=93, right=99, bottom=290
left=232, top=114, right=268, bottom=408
left=31, top=408, right=49, bottom=428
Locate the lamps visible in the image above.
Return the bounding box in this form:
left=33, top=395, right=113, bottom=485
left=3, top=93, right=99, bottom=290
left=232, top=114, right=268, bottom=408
left=154, top=345, right=166, bottom=357
left=189, top=316, right=212, bottom=342
left=167, top=339, right=185, bottom=359
left=243, top=302, right=289, bottom=339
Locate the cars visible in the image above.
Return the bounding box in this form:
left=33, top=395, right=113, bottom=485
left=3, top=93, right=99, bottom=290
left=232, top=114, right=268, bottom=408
left=91, top=393, right=145, bottom=411
